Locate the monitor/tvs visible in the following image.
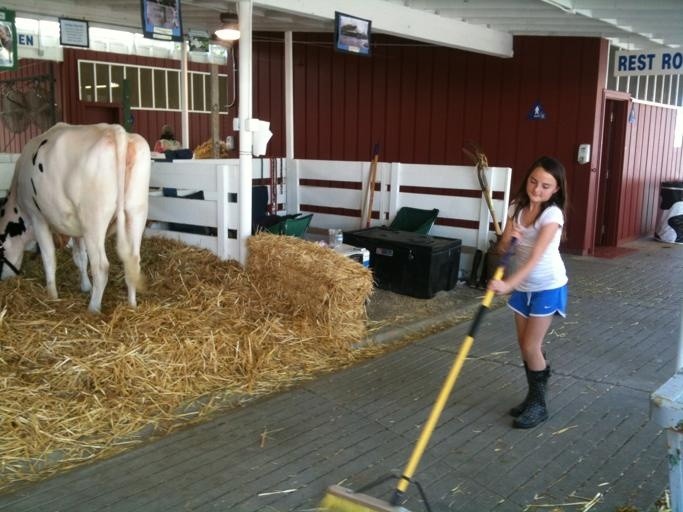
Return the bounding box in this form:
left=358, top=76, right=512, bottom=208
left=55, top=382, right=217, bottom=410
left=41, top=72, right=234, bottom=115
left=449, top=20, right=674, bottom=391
left=140, top=0, right=184, bottom=42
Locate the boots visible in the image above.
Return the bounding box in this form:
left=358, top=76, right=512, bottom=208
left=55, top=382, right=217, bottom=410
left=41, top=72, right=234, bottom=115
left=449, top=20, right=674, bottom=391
left=509, top=352, right=552, bottom=429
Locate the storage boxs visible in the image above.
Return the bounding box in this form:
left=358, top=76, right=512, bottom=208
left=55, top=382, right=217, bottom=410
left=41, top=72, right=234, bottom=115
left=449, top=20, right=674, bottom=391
left=343, top=226, right=462, bottom=299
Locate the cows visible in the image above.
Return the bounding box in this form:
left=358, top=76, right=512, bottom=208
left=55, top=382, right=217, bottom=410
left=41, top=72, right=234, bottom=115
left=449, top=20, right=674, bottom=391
left=0, top=121, right=151, bottom=315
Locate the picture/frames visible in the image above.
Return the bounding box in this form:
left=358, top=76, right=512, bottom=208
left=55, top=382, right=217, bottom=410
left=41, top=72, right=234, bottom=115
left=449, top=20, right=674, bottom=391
left=58, top=17, right=90, bottom=48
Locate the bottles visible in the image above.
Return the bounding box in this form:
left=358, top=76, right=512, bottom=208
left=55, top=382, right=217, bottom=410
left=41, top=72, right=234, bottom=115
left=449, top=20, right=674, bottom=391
left=335, top=229, right=343, bottom=249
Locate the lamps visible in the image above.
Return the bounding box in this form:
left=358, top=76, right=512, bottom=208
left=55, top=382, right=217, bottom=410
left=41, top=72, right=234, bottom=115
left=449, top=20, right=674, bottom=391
left=215, top=13, right=241, bottom=40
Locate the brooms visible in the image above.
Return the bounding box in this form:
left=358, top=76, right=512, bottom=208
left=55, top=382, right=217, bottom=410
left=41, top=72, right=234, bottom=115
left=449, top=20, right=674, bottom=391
left=319, top=266, right=506, bottom=512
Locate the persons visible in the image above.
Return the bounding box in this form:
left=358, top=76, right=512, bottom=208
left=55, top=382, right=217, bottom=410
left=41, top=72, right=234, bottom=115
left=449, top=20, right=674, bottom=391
left=149, top=4, right=165, bottom=27
left=481, top=153, right=569, bottom=431
left=153, top=124, right=182, bottom=154
left=340, top=24, right=360, bottom=44
left=161, top=6, right=177, bottom=30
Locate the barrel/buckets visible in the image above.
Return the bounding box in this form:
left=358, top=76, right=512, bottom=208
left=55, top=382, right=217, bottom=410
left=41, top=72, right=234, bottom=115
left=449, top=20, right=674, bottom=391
left=653, top=180, right=683, bottom=244
